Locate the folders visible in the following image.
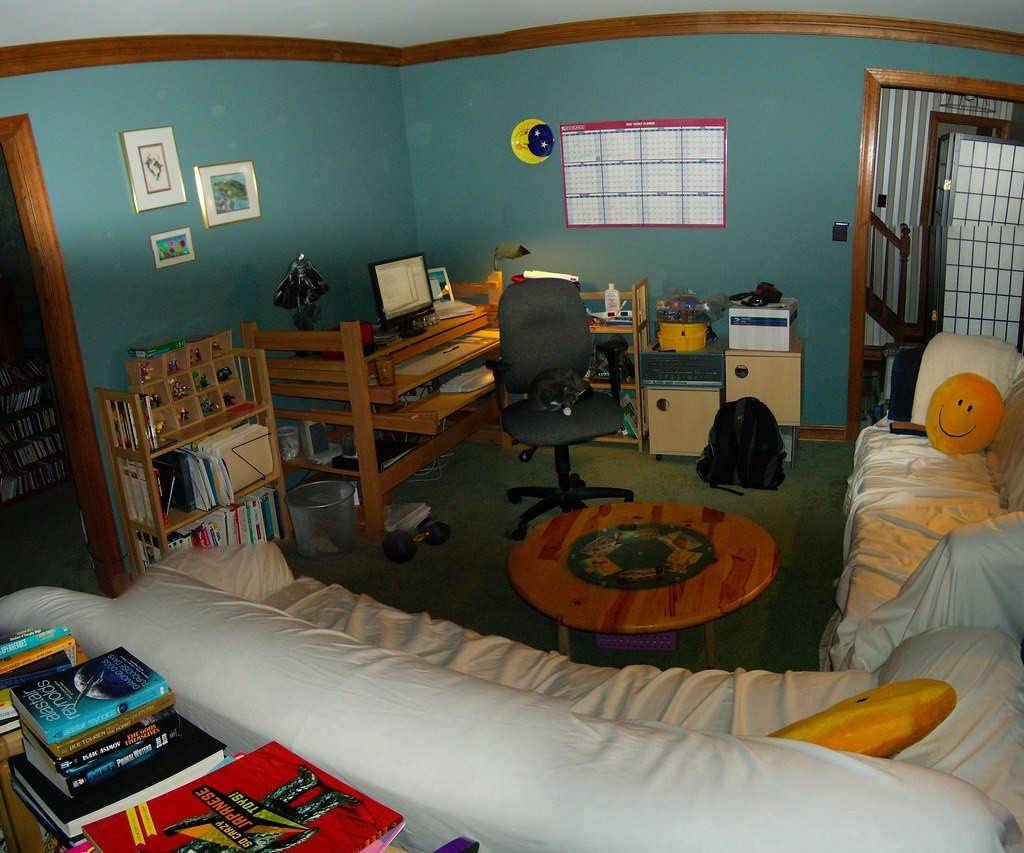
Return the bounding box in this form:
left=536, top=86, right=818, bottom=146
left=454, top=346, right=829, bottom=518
left=333, top=442, right=419, bottom=474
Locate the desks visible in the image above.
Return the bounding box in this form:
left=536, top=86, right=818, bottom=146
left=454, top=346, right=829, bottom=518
left=240, top=270, right=515, bottom=546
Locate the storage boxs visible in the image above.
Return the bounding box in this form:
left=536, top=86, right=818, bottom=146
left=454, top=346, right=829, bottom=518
left=729, top=297, right=797, bottom=352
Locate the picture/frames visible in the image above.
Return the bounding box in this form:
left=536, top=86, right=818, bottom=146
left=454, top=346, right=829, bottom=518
left=193, top=160, right=262, bottom=230
left=150, top=227, right=196, bottom=270
left=118, top=127, right=187, bottom=214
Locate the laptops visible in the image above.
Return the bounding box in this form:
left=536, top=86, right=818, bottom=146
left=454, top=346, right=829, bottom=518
left=426, top=267, right=477, bottom=316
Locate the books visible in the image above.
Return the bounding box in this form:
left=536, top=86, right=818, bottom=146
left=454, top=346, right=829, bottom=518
left=0, top=359, right=66, bottom=502
left=137, top=487, right=282, bottom=570
left=9, top=646, right=228, bottom=848
left=128, top=335, right=185, bottom=358
left=617, top=394, right=648, bottom=440
left=104, top=394, right=158, bottom=451
left=360, top=501, right=430, bottom=530
left=67, top=742, right=406, bottom=853
left=120, top=424, right=295, bottom=524
left=425, top=300, right=476, bottom=320
left=0, top=625, right=77, bottom=734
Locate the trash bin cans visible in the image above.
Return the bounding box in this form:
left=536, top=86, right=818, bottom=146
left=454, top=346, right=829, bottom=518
left=284, top=479, right=357, bottom=562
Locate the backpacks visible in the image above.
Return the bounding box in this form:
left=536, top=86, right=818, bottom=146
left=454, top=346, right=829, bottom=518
left=695, top=396, right=788, bottom=495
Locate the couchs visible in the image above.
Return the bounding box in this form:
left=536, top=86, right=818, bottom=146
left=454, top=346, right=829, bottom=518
left=0, top=544, right=1024, bottom=853
left=817, top=333, right=1024, bottom=675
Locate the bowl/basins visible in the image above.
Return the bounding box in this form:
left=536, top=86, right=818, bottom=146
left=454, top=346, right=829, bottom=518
left=657, top=322, right=707, bottom=351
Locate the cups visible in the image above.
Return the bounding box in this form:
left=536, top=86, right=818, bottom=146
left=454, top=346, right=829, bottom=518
left=376, top=356, right=395, bottom=385
left=277, top=426, right=299, bottom=461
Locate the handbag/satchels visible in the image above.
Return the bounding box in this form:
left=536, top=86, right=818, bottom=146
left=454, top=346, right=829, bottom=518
left=530, top=368, right=593, bottom=413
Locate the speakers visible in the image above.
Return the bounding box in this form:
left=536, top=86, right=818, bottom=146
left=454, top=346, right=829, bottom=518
left=297, top=420, right=330, bottom=457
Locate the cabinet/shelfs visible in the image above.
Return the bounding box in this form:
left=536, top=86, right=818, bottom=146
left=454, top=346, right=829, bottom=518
left=94, top=329, right=293, bottom=584
left=725, top=337, right=804, bottom=468
left=0, top=359, right=74, bottom=512
left=646, top=386, right=722, bottom=461
left=580, top=276, right=650, bottom=452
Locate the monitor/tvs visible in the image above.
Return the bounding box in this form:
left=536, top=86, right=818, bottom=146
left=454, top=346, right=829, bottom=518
left=369, top=251, right=436, bottom=339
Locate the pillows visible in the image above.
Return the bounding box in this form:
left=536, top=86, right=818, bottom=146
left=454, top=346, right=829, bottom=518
left=767, top=679, right=956, bottom=757
left=924, top=373, right=1004, bottom=456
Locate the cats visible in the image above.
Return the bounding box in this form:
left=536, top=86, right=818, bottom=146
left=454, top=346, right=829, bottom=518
left=528, top=368, right=593, bottom=416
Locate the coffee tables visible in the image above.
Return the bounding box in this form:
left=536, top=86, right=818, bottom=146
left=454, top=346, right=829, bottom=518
left=507, top=502, right=779, bottom=669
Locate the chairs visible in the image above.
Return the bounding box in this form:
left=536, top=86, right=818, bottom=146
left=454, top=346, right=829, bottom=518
left=485, top=278, right=634, bottom=540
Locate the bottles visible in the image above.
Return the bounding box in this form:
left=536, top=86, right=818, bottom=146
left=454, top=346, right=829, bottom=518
left=605, top=283, right=620, bottom=312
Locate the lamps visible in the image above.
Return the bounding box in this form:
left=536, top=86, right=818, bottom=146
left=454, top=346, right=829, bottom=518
left=940, top=93, right=997, bottom=113
left=493, top=241, right=531, bottom=271
left=274, top=253, right=330, bottom=356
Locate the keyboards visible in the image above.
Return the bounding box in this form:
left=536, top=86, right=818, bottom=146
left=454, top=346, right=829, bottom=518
left=439, top=366, right=496, bottom=393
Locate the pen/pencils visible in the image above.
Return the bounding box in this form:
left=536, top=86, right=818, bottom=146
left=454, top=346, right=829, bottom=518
left=443, top=346, right=460, bottom=355
